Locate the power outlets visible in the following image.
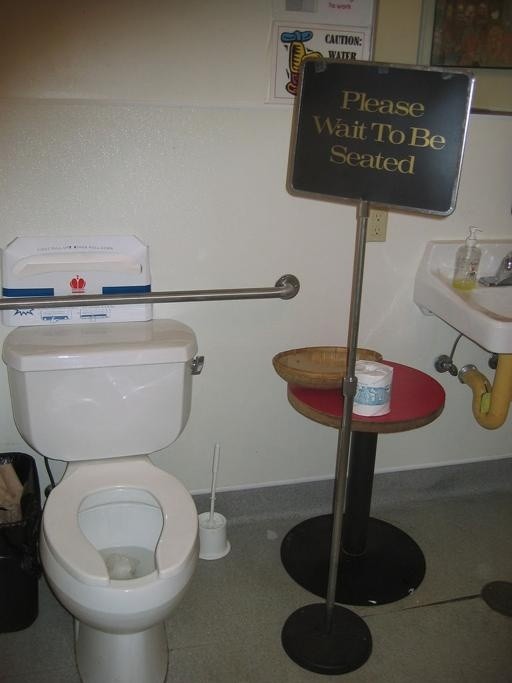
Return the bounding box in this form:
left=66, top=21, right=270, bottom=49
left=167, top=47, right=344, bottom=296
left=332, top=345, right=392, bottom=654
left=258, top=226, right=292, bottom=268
left=363, top=207, right=388, bottom=245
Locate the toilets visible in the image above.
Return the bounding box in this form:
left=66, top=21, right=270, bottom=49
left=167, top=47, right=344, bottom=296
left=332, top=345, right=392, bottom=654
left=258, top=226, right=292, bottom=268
left=0, top=319, right=205, bottom=683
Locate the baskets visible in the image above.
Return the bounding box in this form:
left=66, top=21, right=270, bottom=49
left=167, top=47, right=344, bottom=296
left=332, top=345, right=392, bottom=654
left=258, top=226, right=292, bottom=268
left=273, top=346, right=382, bottom=390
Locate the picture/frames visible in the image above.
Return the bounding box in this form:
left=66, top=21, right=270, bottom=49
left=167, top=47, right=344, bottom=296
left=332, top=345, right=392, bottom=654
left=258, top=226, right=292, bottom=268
left=415, top=1, right=511, bottom=116
left=265, top=20, right=371, bottom=105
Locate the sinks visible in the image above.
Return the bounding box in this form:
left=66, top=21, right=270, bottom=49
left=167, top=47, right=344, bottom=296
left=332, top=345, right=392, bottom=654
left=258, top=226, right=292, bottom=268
left=410, top=236, right=512, bottom=359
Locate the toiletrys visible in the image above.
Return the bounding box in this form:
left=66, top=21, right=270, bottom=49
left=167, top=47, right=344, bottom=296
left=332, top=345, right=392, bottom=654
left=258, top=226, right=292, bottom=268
left=452, top=226, right=483, bottom=290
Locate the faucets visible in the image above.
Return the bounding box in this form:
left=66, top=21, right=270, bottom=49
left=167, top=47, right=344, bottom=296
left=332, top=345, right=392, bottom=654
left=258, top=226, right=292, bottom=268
left=479, top=253, right=512, bottom=287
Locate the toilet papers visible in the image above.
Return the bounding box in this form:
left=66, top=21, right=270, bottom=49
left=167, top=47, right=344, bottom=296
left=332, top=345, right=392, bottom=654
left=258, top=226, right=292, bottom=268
left=352, top=359, right=392, bottom=416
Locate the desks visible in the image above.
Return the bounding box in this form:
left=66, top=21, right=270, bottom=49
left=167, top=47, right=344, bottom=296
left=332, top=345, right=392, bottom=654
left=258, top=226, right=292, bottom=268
left=279, top=357, right=445, bottom=607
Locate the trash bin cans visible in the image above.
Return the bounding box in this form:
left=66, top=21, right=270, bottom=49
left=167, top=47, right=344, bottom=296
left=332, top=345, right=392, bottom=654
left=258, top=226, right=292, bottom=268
left=0, top=452, right=41, bottom=634
